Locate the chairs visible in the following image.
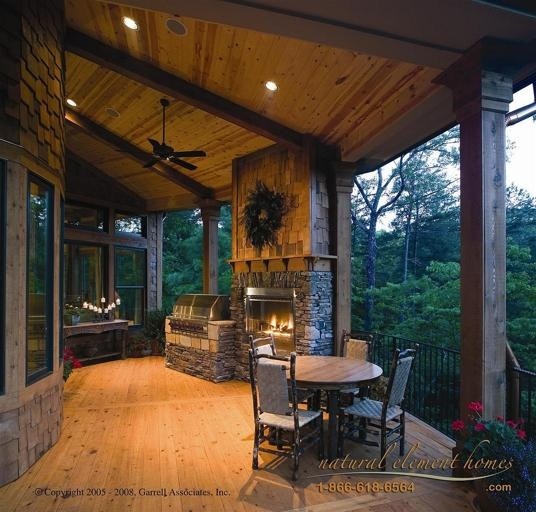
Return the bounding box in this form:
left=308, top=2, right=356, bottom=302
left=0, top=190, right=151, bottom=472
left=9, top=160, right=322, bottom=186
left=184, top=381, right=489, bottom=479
left=244, top=327, right=421, bottom=482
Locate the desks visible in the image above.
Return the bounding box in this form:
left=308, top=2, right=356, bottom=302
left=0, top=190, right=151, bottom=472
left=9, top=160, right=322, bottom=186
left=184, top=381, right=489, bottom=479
left=62, top=319, right=128, bottom=369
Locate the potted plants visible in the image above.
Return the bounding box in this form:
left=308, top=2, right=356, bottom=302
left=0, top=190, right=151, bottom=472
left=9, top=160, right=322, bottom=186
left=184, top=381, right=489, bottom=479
left=141, top=305, right=172, bottom=357
left=65, top=303, right=84, bottom=326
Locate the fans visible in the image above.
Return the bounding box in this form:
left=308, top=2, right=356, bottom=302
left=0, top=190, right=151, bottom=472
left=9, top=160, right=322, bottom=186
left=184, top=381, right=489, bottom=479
left=114, top=97, right=208, bottom=172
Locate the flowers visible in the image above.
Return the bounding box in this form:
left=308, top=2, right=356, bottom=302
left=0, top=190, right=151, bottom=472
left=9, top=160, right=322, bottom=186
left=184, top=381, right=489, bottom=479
left=62, top=346, right=81, bottom=382
left=450, top=400, right=536, bottom=511
